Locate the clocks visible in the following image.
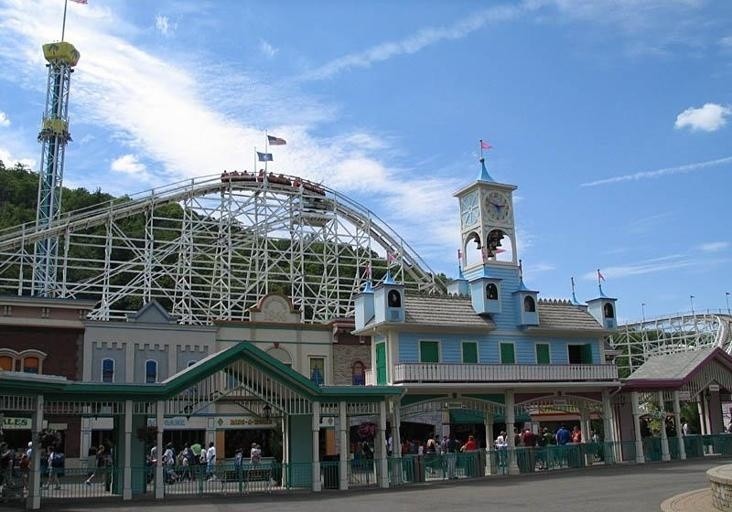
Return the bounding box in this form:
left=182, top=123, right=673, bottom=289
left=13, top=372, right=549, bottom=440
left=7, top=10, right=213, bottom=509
left=482, top=189, right=512, bottom=224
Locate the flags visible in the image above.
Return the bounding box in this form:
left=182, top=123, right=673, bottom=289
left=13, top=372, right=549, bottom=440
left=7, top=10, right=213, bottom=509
left=268, top=135, right=286, bottom=145
left=481, top=142, right=492, bottom=148
left=71, top=0, right=87, bottom=4
left=258, top=152, right=272, bottom=161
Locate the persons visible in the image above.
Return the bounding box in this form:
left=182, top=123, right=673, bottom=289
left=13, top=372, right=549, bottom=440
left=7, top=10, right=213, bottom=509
left=497, top=425, right=581, bottom=470
left=681, top=417, right=687, bottom=436
left=234, top=447, right=244, bottom=469
left=86, top=444, right=106, bottom=486
left=386, top=432, right=481, bottom=479
left=1, top=437, right=61, bottom=494
left=259, top=169, right=283, bottom=178
left=250, top=442, right=261, bottom=464
left=149, top=439, right=217, bottom=483
left=591, top=429, right=600, bottom=462
left=221, top=169, right=254, bottom=176
